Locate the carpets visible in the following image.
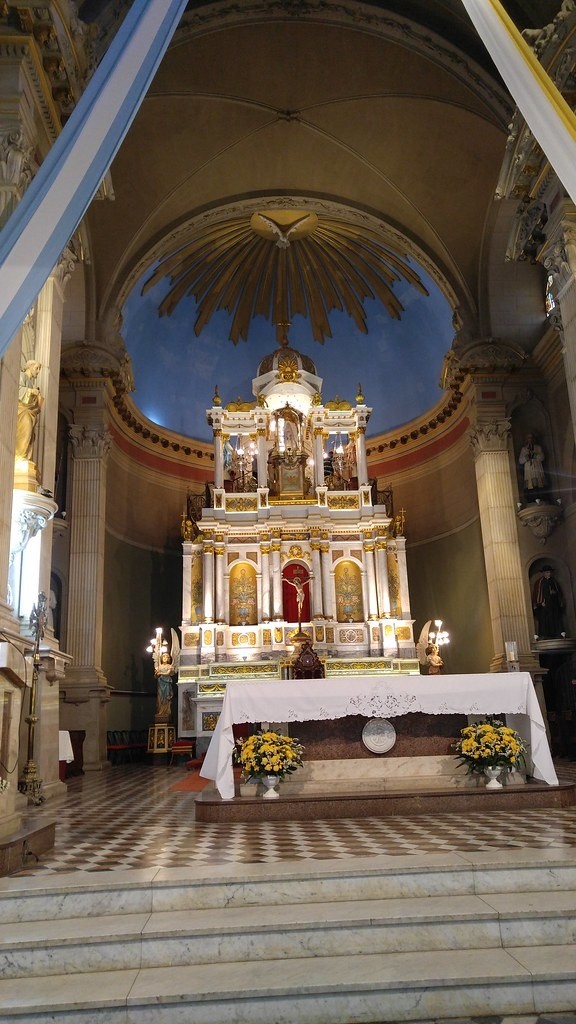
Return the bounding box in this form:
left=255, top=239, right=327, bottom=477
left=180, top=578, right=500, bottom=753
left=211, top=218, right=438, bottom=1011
left=166, top=763, right=243, bottom=793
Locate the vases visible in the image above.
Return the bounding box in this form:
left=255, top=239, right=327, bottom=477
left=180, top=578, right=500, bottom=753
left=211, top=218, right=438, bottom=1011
left=483, top=765, right=505, bottom=790
left=239, top=783, right=257, bottom=797
left=261, top=773, right=282, bottom=800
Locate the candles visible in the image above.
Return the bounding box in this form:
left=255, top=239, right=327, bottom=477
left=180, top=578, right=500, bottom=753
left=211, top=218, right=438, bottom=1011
left=505, top=642, right=519, bottom=661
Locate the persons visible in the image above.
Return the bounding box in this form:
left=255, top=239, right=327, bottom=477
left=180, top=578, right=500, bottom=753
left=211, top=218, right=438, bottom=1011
left=324, top=451, right=334, bottom=476
left=252, top=455, right=257, bottom=484
left=15, top=360, right=44, bottom=464
left=532, top=566, right=566, bottom=640
left=427, top=646, right=445, bottom=675
left=553, top=658, right=576, bottom=762
left=393, top=516, right=406, bottom=534
left=267, top=444, right=278, bottom=489
left=518, top=433, right=550, bottom=502
left=284, top=578, right=313, bottom=608
left=182, top=520, right=193, bottom=541
left=154, top=653, right=174, bottom=716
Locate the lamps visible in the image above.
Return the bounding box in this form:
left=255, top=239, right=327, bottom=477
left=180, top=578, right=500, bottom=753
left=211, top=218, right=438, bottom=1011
left=427, top=619, right=452, bottom=646
left=332, top=433, right=345, bottom=463
left=235, top=645, right=253, bottom=662
left=146, top=627, right=169, bottom=674
left=236, top=438, right=258, bottom=480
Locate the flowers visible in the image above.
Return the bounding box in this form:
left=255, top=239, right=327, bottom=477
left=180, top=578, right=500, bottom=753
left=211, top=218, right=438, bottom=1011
left=450, top=720, right=530, bottom=784
left=232, top=729, right=306, bottom=785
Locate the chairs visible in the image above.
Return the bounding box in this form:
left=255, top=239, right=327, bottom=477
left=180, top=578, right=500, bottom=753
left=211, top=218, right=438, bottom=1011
left=106, top=730, right=148, bottom=767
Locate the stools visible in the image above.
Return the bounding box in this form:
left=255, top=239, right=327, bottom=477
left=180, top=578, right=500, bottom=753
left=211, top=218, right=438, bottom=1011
left=168, top=741, right=193, bottom=770
